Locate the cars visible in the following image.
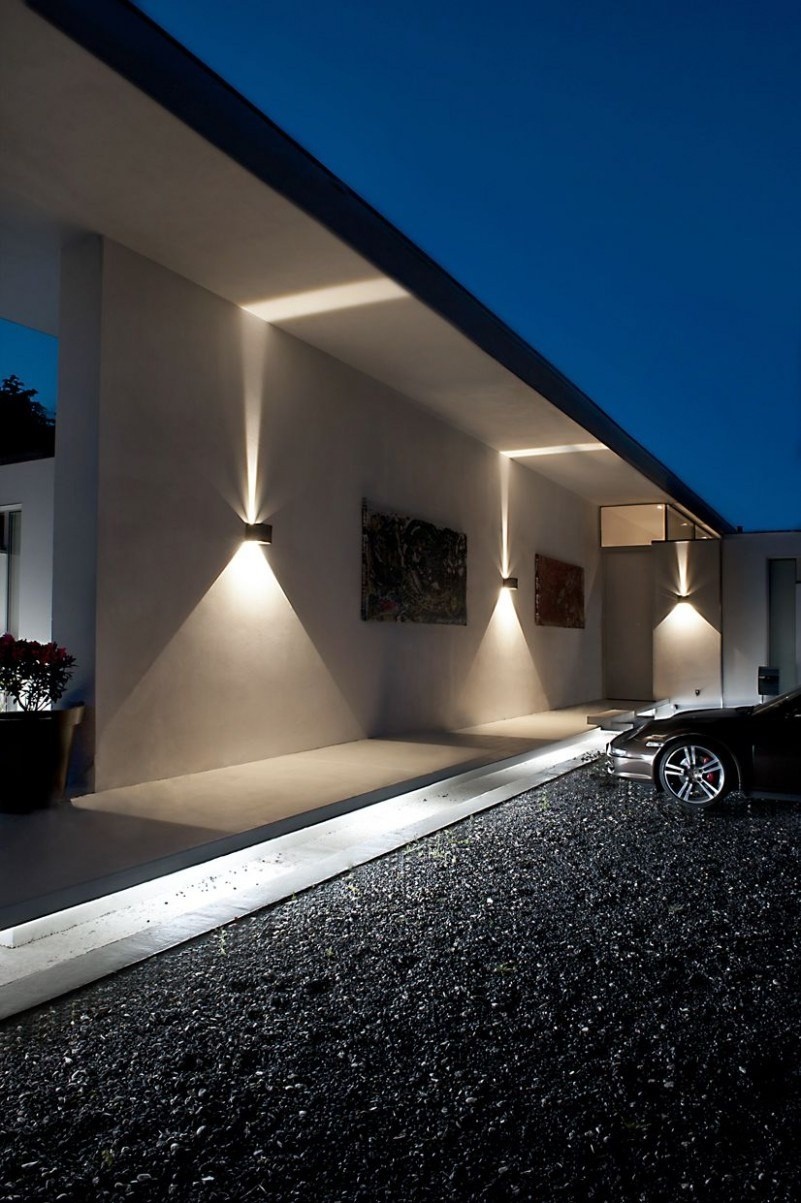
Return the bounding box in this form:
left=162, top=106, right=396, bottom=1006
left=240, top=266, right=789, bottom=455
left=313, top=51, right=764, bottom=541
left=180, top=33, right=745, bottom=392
left=607, top=687, right=801, bottom=806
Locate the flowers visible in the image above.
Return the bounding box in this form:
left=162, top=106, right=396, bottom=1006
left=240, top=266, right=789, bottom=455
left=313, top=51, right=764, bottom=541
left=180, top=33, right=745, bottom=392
left=0, top=633, right=81, bottom=716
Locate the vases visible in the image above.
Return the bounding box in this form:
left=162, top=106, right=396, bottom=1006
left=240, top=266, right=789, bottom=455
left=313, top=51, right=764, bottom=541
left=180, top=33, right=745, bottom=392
left=0, top=703, right=88, bottom=813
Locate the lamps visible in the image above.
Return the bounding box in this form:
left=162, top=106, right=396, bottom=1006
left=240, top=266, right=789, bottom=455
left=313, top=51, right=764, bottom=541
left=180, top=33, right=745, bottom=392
left=245, top=522, right=274, bottom=546
left=677, top=592, right=692, bottom=599
left=502, top=577, right=519, bottom=588
